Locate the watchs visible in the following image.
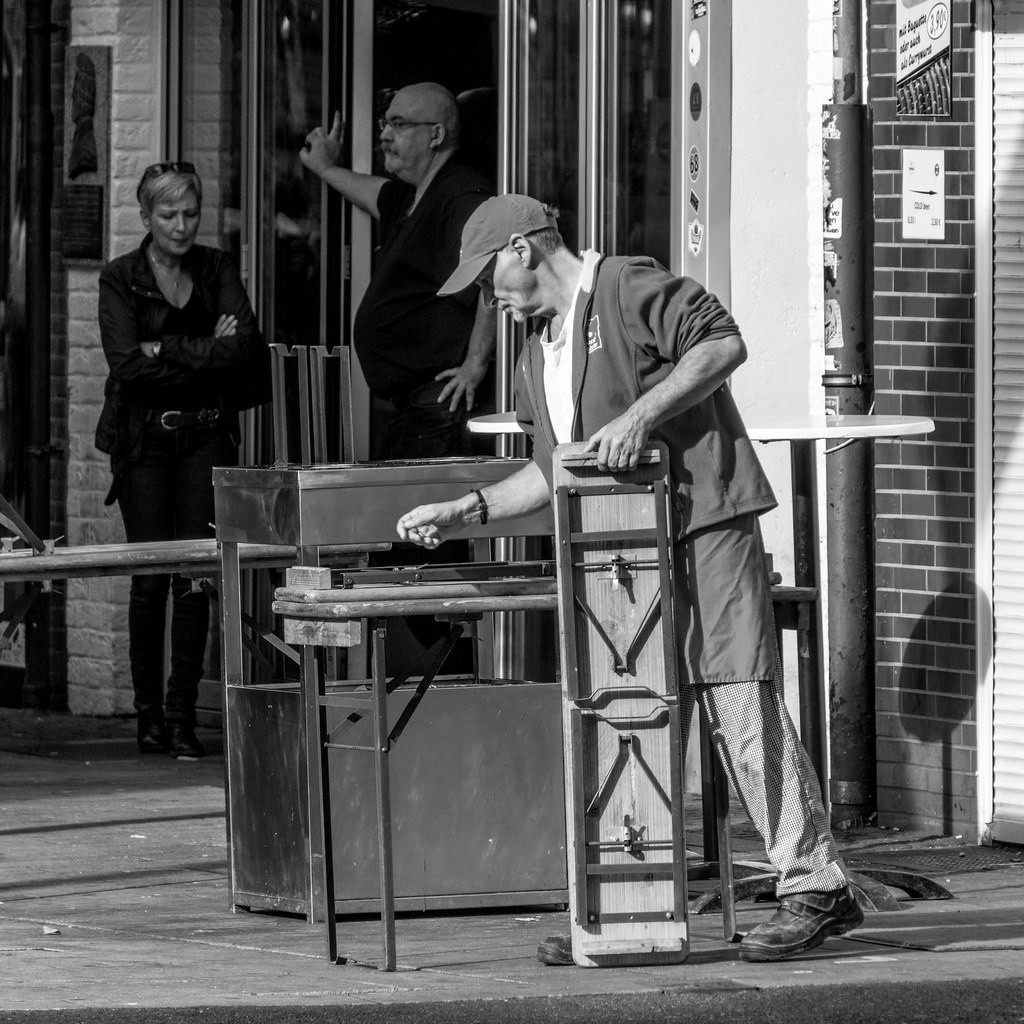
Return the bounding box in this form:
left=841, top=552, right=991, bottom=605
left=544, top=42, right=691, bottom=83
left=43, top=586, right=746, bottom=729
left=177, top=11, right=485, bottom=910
left=152, top=341, right=161, bottom=358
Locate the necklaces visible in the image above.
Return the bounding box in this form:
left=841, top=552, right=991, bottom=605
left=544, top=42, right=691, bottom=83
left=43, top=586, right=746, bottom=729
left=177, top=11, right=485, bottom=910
left=149, top=248, right=179, bottom=288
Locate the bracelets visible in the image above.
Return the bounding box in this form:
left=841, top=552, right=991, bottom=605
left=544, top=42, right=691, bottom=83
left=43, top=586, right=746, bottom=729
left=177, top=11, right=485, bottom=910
left=470, top=488, right=488, bottom=524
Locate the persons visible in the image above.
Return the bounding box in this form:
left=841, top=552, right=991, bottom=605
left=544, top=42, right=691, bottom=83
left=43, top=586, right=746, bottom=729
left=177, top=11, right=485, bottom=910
left=95, top=161, right=270, bottom=756
left=300, top=84, right=495, bottom=672
left=397, top=193, right=864, bottom=963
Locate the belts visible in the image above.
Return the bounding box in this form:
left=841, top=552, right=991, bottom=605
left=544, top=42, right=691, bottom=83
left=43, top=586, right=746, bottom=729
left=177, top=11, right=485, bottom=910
left=136, top=409, right=221, bottom=433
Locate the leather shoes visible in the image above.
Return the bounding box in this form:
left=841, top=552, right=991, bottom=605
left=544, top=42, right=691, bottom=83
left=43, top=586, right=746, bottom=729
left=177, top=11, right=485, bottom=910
left=737, top=888, right=865, bottom=962
left=137, top=708, right=168, bottom=751
left=166, top=720, right=205, bottom=757
left=538, top=933, right=574, bottom=965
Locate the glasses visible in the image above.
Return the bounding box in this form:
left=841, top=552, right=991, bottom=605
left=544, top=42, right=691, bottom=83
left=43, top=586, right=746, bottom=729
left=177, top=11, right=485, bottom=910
left=379, top=119, right=436, bottom=134
left=136, top=162, right=195, bottom=203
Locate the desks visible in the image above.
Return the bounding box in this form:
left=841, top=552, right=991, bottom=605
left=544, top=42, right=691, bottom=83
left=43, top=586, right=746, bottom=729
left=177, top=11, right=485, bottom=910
left=0, top=536, right=392, bottom=687
left=467, top=411, right=955, bottom=915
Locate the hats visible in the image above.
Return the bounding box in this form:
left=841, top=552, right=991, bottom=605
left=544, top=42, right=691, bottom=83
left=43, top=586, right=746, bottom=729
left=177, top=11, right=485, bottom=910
left=436, top=193, right=558, bottom=296
